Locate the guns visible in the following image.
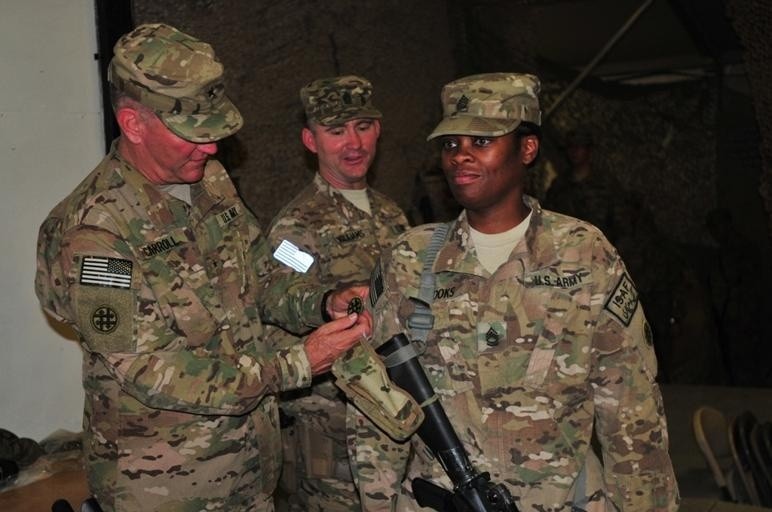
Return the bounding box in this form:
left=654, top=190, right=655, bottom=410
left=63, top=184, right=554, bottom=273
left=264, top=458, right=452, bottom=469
left=374, top=332, right=517, bottom=511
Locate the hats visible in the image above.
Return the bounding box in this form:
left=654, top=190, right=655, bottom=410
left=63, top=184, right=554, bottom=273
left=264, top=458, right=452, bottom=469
left=106, top=23, right=244, bottom=144
left=300, top=75, right=384, bottom=126
left=426, top=72, right=543, bottom=142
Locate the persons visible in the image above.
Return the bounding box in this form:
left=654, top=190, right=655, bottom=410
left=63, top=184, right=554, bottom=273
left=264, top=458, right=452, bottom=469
left=346, top=72, right=682, bottom=512
left=268, top=71, right=413, bottom=512
left=34, top=22, right=371, bottom=512
left=415, top=105, right=449, bottom=224
left=550, top=136, right=631, bottom=250
